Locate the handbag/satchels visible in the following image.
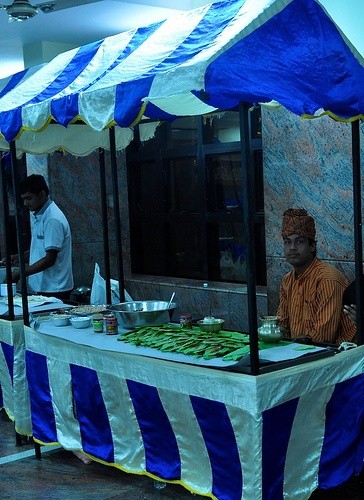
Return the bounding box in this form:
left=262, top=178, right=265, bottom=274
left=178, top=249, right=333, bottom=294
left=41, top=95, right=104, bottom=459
left=90, top=263, right=133, bottom=307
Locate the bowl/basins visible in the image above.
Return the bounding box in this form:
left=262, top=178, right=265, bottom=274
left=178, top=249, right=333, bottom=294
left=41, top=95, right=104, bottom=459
left=69, top=317, right=93, bottom=329
left=50, top=314, right=71, bottom=327
left=1, top=283, right=16, bottom=297
left=197, top=319, right=225, bottom=333
left=104, top=301, right=181, bottom=328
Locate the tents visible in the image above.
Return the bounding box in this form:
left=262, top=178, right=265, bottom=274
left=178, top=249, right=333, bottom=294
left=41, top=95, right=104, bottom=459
left=0, top=0, right=364, bottom=375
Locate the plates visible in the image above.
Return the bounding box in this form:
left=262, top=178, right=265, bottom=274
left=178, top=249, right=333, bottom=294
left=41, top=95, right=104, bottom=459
left=65, top=305, right=111, bottom=317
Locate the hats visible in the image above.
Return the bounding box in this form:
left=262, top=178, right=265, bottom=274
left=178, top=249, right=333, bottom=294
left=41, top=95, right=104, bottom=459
left=281, top=209, right=316, bottom=241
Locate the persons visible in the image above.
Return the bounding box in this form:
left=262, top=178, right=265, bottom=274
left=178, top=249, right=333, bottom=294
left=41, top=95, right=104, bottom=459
left=275, top=208, right=357, bottom=348
left=0, top=174, right=74, bottom=301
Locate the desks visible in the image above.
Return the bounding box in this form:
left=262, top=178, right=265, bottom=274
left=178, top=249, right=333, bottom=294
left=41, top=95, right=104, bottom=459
left=0, top=293, right=364, bottom=500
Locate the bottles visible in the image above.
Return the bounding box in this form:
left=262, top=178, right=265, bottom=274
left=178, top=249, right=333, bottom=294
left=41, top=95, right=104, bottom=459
left=180, top=314, right=192, bottom=330
left=257, top=315, right=284, bottom=344
left=93, top=313, right=118, bottom=335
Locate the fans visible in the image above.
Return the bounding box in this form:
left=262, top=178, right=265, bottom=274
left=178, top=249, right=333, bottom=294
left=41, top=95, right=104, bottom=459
left=0, top=0, right=102, bottom=24
